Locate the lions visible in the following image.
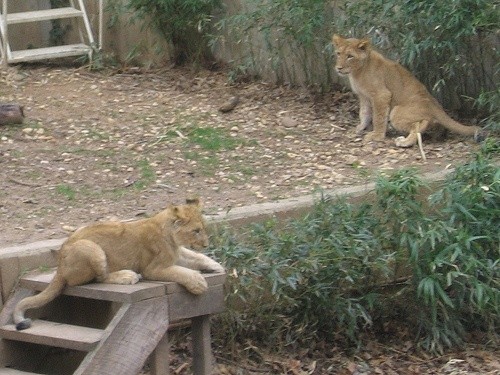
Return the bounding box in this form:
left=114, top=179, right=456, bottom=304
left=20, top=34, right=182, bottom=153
left=14, top=198, right=225, bottom=330
left=333, top=33, right=484, bottom=147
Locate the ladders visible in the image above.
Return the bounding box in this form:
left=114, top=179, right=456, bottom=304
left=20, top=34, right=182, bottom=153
left=0, top=0, right=103, bottom=66
